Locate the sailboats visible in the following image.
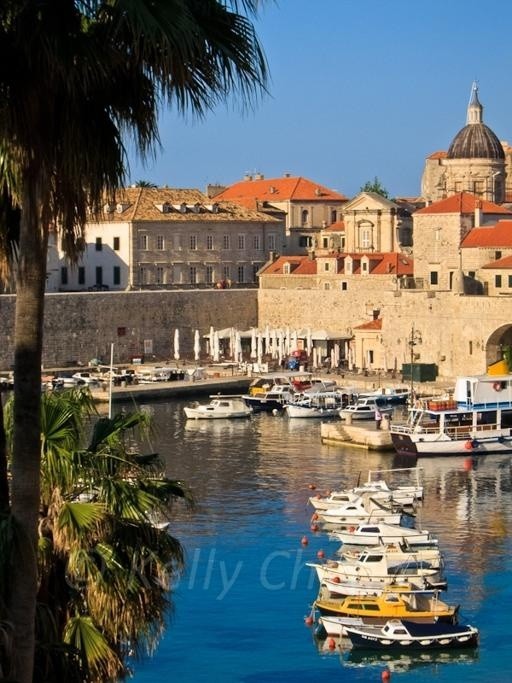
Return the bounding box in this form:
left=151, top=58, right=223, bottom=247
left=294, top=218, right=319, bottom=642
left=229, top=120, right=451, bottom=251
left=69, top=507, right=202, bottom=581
left=70, top=340, right=172, bottom=534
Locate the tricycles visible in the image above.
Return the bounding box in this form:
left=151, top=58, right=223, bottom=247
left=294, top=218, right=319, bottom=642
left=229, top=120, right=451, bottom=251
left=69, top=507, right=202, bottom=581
left=286, top=354, right=300, bottom=371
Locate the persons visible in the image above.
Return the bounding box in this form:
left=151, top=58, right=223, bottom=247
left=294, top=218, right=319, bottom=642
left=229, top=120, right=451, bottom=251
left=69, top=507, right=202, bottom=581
left=374, top=407, right=382, bottom=430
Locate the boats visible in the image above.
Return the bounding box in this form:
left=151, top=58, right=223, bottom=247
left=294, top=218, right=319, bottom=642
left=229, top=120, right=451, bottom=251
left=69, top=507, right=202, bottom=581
left=391, top=449, right=511, bottom=522
left=0, top=349, right=206, bottom=394
left=341, top=646, right=480, bottom=669
left=388, top=358, right=512, bottom=456
left=182, top=370, right=411, bottom=420
left=307, top=466, right=482, bottom=654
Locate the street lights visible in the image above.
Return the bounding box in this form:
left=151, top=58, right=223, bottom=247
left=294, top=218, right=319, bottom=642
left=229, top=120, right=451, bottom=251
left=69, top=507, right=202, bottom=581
left=396, top=321, right=423, bottom=415
